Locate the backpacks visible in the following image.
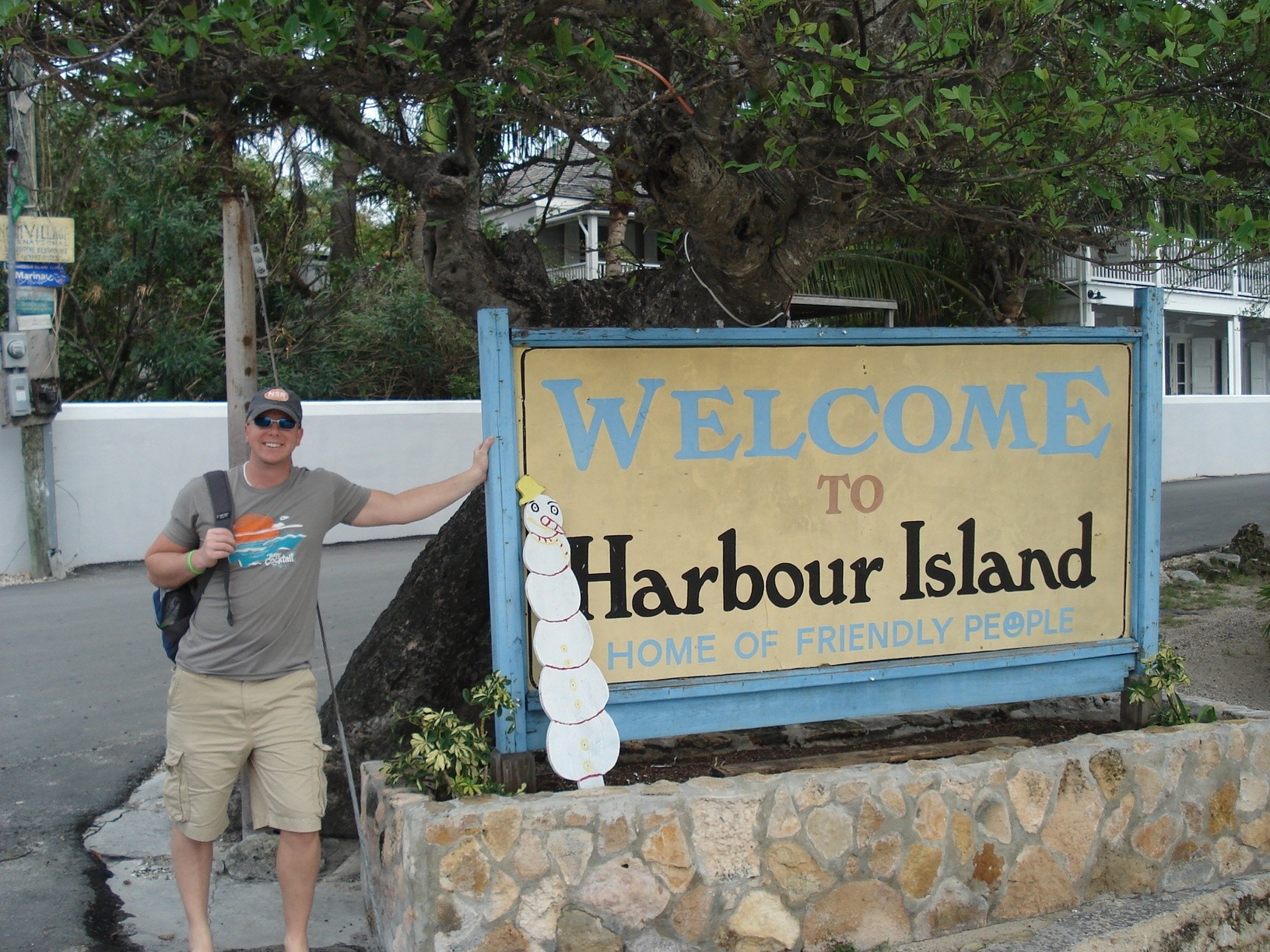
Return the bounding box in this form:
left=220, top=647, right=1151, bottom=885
left=153, top=471, right=234, bottom=664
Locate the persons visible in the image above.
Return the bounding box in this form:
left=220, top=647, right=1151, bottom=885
left=140, top=384, right=496, bottom=952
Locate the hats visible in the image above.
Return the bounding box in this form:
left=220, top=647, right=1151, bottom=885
left=247, top=387, right=302, bottom=428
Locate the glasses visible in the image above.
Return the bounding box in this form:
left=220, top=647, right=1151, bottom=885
left=251, top=416, right=297, bottom=430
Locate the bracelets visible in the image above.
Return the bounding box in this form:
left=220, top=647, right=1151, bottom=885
left=187, top=549, right=206, bottom=576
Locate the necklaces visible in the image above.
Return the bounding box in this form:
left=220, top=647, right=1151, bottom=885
left=243, top=460, right=253, bottom=487
